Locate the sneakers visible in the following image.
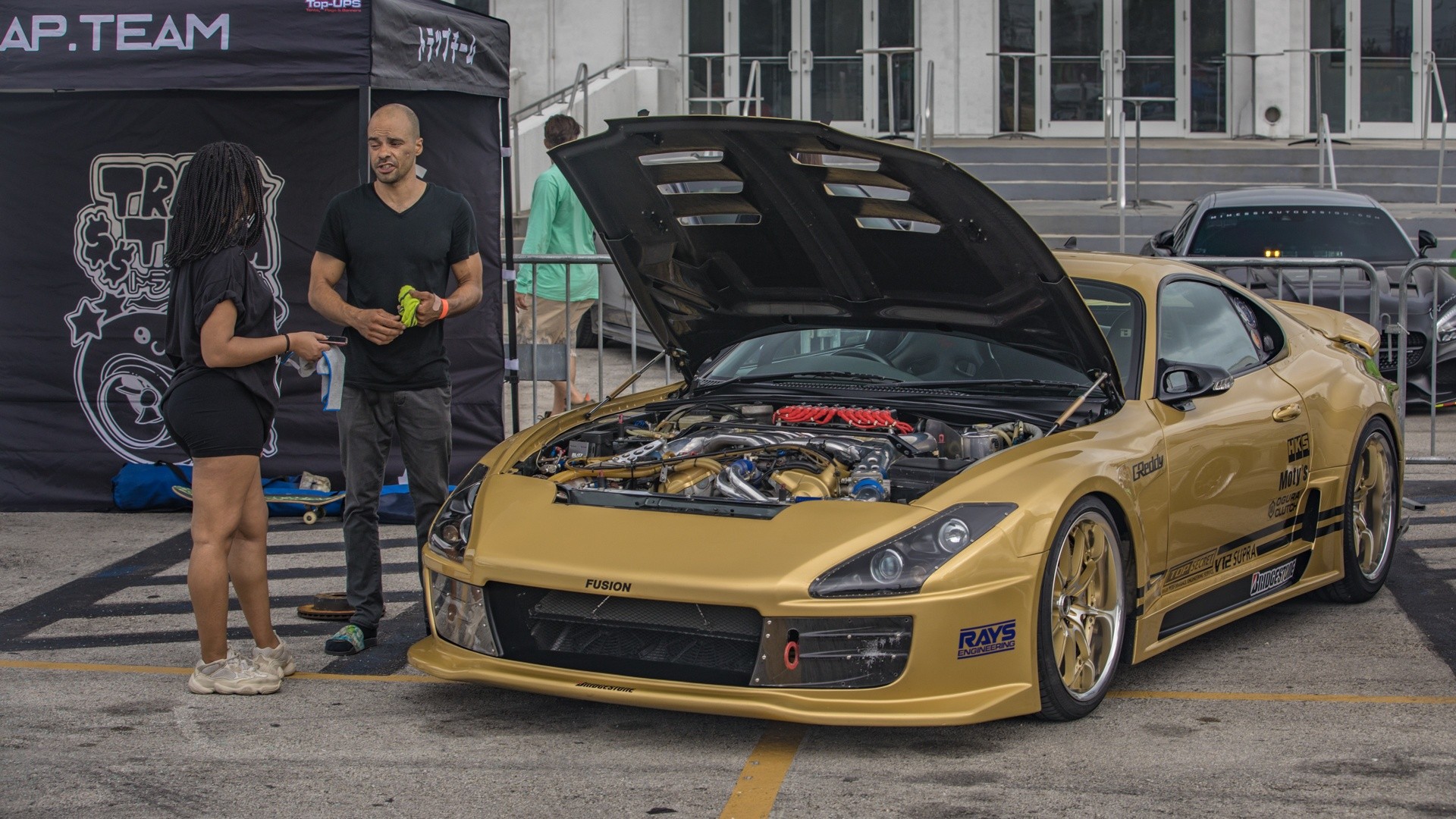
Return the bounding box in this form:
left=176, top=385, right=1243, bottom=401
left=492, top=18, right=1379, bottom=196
left=537, top=411, right=552, bottom=422
left=188, top=644, right=282, bottom=696
left=563, top=393, right=597, bottom=411
left=252, top=630, right=296, bottom=676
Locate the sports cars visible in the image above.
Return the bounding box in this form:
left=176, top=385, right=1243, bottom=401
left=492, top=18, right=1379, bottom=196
left=1139, top=186, right=1456, bottom=410
left=407, top=114, right=1424, bottom=726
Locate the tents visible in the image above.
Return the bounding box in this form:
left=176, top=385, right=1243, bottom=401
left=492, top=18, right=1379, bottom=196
left=0, top=1, right=515, bottom=515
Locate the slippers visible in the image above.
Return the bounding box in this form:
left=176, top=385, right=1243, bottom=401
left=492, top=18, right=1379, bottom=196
left=324, top=623, right=377, bottom=656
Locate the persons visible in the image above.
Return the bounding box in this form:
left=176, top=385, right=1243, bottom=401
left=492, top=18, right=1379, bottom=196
left=159, top=142, right=332, bottom=695
left=309, top=103, right=484, bottom=656
left=516, top=114, right=598, bottom=422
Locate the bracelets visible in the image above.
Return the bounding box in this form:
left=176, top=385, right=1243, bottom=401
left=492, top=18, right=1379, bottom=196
left=437, top=298, right=448, bottom=319
left=282, top=333, right=291, bottom=353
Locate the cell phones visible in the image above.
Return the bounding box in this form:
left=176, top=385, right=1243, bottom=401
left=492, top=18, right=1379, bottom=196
left=317, top=335, right=349, bottom=346
